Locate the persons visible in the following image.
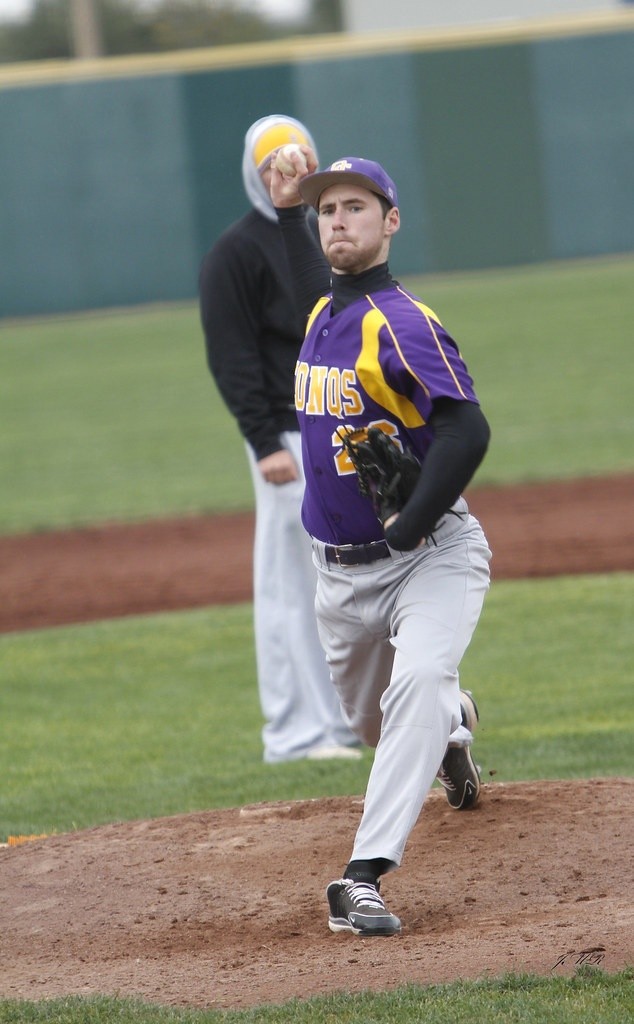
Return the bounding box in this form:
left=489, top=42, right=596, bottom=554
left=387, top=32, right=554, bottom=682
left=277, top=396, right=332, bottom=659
left=197, top=113, right=361, bottom=758
left=269, top=145, right=493, bottom=935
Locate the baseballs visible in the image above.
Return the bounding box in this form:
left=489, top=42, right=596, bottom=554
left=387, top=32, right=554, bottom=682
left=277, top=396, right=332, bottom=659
left=276, top=144, right=306, bottom=174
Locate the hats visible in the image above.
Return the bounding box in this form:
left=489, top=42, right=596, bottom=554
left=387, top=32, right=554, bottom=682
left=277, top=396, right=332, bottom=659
left=298, top=156, right=400, bottom=213
left=255, top=123, right=306, bottom=174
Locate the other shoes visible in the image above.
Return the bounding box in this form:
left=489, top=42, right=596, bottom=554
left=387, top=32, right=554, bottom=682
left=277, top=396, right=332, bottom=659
left=259, top=745, right=364, bottom=762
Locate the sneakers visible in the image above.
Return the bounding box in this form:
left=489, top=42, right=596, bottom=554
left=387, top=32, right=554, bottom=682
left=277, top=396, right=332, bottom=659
left=325, top=878, right=402, bottom=937
left=439, top=693, right=481, bottom=809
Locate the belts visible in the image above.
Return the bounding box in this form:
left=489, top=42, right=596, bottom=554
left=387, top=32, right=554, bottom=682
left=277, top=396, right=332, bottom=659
left=310, top=541, right=389, bottom=567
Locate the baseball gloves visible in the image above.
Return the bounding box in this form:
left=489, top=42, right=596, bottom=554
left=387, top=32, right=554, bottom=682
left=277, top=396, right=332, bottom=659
left=334, top=422, right=467, bottom=547
